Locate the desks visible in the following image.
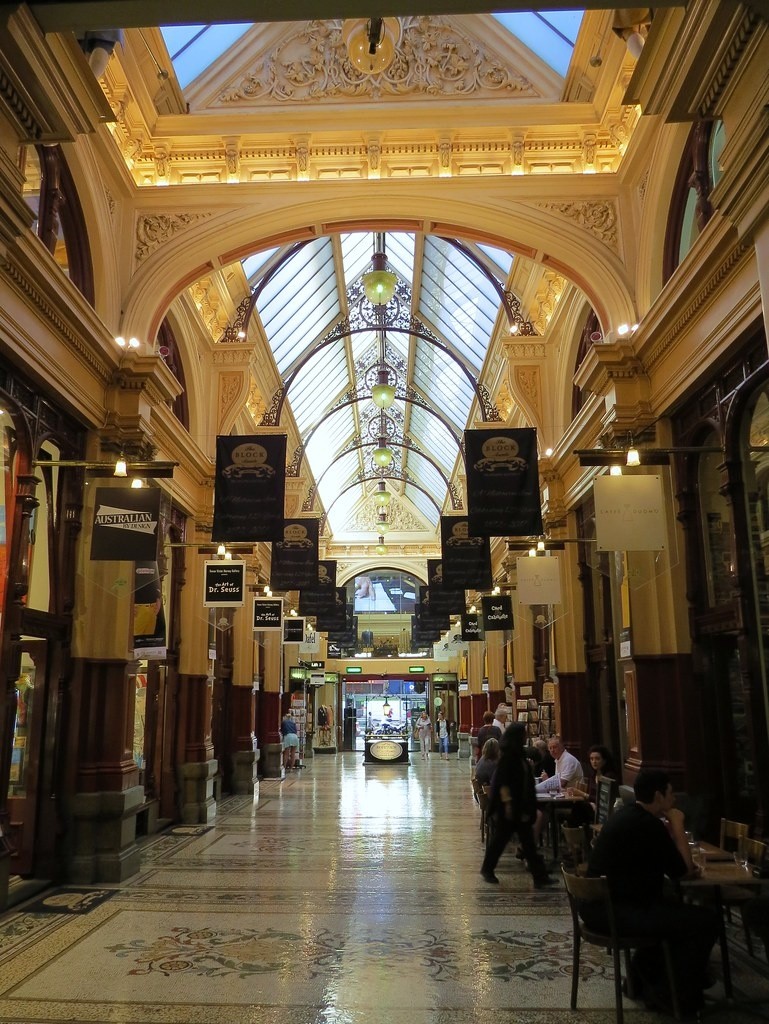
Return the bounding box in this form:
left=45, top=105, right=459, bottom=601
left=539, top=790, right=587, bottom=863
left=690, top=840, right=769, bottom=1011
left=362, top=735, right=411, bottom=765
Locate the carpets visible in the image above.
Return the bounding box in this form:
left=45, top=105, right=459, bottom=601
left=161, top=825, right=216, bottom=837
left=20, top=888, right=121, bottom=915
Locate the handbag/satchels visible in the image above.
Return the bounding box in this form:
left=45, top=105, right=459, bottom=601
left=414, top=726, right=419, bottom=739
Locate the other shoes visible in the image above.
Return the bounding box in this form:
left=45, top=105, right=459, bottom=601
left=534, top=875, right=560, bottom=889
left=480, top=868, right=499, bottom=883
left=440, top=757, right=442, bottom=759
left=422, top=753, right=429, bottom=760
left=445, top=755, right=449, bottom=760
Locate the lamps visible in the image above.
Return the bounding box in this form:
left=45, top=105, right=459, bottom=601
left=455, top=620, right=461, bottom=627
left=469, top=604, right=477, bottom=614
left=262, top=577, right=273, bottom=597
left=114, top=440, right=135, bottom=477
left=609, top=466, right=622, bottom=475
left=291, top=608, right=313, bottom=630
left=383, top=695, right=391, bottom=715
left=371, top=304, right=397, bottom=409
left=491, top=577, right=501, bottom=596
left=362, top=233, right=398, bottom=304
left=114, top=336, right=140, bottom=351
left=376, top=534, right=388, bottom=556
left=217, top=542, right=233, bottom=561
left=623, top=427, right=641, bottom=467
left=376, top=506, right=391, bottom=535
left=343, top=16, right=403, bottom=74
left=374, top=466, right=392, bottom=507
left=529, top=540, right=545, bottom=557
left=373, top=408, right=393, bottom=466
left=231, top=331, right=247, bottom=340
left=131, top=476, right=145, bottom=489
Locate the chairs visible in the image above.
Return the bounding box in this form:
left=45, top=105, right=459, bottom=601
left=718, top=817, right=754, bottom=958
left=717, top=835, right=769, bottom=964
left=470, top=778, right=492, bottom=844
left=553, top=781, right=589, bottom=859
left=558, top=862, right=682, bottom=1024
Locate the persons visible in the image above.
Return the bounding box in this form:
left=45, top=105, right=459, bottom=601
left=436, top=711, right=449, bottom=759
left=417, top=712, right=432, bottom=760
left=474, top=708, right=618, bottom=888
left=282, top=714, right=298, bottom=773
left=580, top=769, right=717, bottom=1005
left=368, top=712, right=374, bottom=727
left=355, top=576, right=375, bottom=600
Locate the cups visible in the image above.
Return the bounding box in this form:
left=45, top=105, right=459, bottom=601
left=568, top=788, right=573, bottom=797
left=538, top=777, right=543, bottom=783
left=693, top=855, right=706, bottom=877
left=685, top=831, right=694, bottom=846
left=534, top=777, right=539, bottom=785
left=733, top=852, right=748, bottom=871
